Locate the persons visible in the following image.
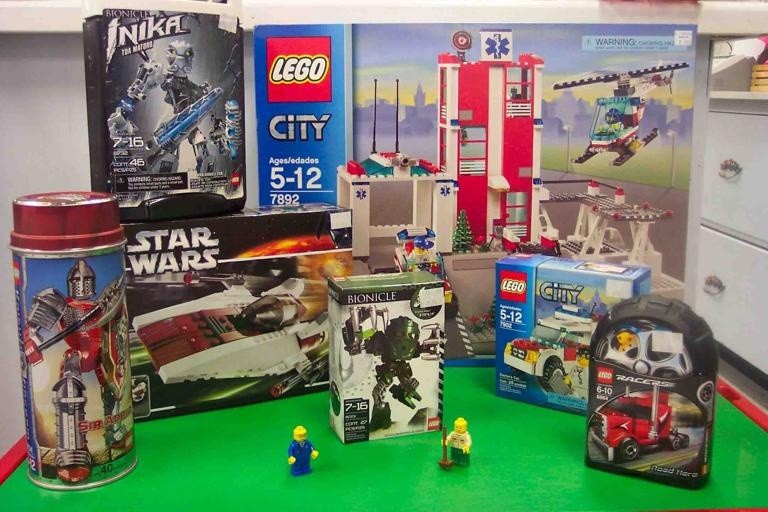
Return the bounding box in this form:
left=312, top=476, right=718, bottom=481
left=288, top=425, right=318, bottom=477
left=441, top=417, right=472, bottom=467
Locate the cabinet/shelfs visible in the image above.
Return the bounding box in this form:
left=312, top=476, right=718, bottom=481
left=692, top=91, right=768, bottom=377
